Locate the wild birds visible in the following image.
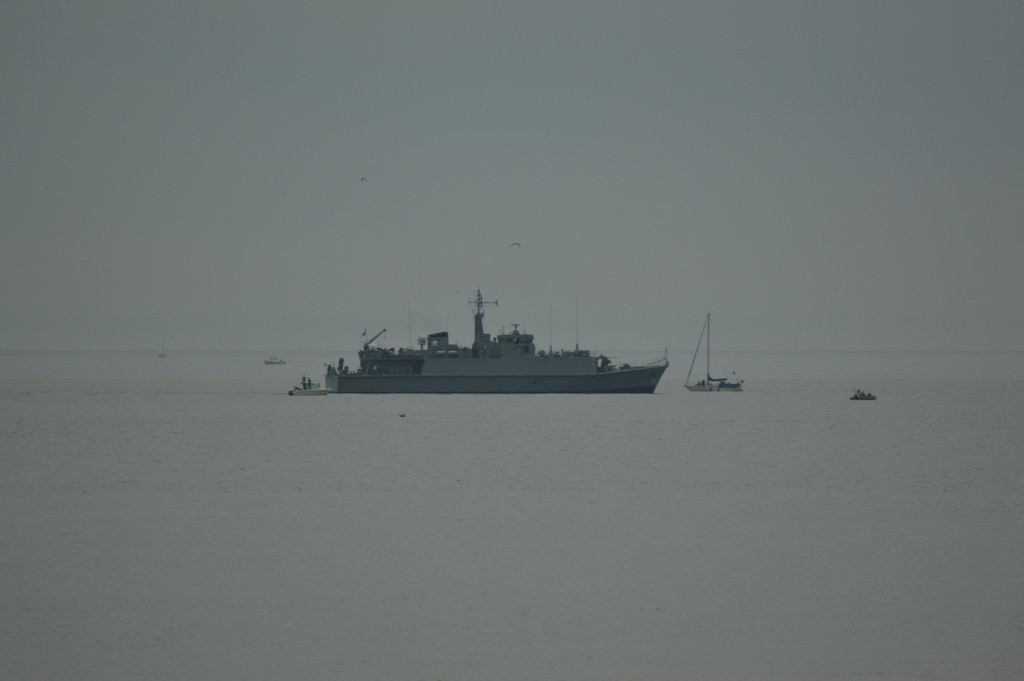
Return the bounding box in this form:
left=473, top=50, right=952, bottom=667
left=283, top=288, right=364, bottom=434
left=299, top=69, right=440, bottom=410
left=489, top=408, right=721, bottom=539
left=360, top=177, right=368, bottom=183
left=399, top=413, right=406, bottom=417
left=510, top=242, right=522, bottom=248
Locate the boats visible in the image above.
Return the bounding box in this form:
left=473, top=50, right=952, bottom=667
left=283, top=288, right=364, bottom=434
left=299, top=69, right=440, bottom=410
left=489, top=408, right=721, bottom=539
left=264, top=357, right=286, bottom=365
left=849, top=389, right=877, bottom=401
left=326, top=288, right=670, bottom=394
left=289, top=377, right=332, bottom=396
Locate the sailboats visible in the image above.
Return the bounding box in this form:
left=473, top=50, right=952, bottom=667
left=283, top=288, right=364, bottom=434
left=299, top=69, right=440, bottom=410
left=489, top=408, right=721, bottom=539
left=682, top=313, right=744, bottom=392
